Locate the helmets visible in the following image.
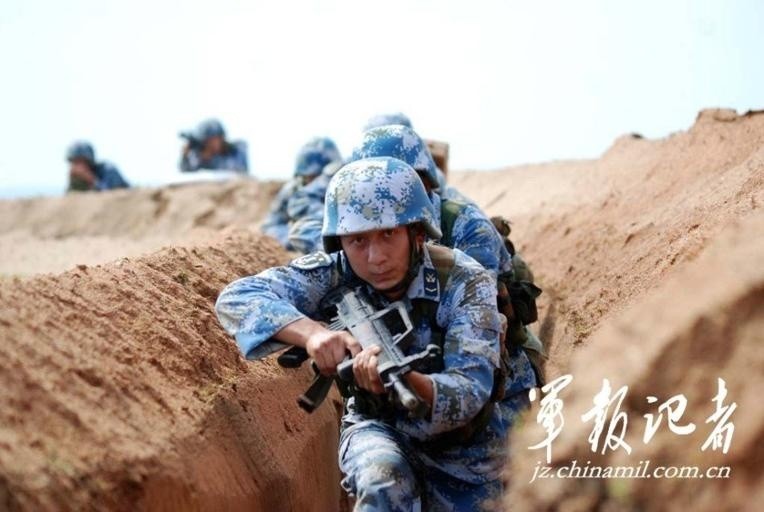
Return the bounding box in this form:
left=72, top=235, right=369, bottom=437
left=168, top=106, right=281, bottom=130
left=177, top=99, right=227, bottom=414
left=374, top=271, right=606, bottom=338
left=65, top=141, right=94, bottom=162
left=295, top=135, right=340, bottom=179
left=352, top=111, right=440, bottom=191
left=193, top=120, right=223, bottom=139
left=322, top=158, right=443, bottom=255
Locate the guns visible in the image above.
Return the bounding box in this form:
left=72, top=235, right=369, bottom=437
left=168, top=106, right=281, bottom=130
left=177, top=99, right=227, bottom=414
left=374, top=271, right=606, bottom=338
left=276, top=288, right=440, bottom=416
left=180, top=132, right=207, bottom=156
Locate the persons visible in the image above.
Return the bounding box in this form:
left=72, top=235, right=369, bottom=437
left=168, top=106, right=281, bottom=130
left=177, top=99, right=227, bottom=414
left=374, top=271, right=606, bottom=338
left=284, top=109, right=445, bottom=222
left=285, top=122, right=540, bottom=511
left=255, top=137, right=343, bottom=255
left=63, top=139, right=129, bottom=190
left=213, top=156, right=515, bottom=512
left=177, top=119, right=248, bottom=173
